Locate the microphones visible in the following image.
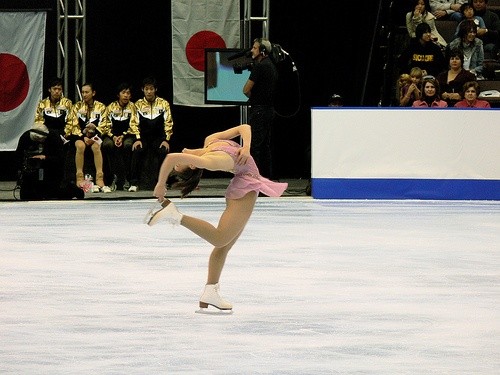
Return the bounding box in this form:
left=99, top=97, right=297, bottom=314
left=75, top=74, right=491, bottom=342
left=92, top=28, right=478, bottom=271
left=228, top=51, right=247, bottom=60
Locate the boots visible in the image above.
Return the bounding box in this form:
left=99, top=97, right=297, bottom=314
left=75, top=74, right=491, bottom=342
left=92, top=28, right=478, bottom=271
left=194, top=280, right=233, bottom=314
left=145, top=196, right=183, bottom=226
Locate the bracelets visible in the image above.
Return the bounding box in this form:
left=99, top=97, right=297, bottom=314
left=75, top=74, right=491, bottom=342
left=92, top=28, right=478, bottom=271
left=486, top=29, right=487, bottom=32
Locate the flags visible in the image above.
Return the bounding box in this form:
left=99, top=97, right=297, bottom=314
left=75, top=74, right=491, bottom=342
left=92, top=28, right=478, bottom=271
left=0, top=12, right=47, bottom=151
left=171, top=0, right=240, bottom=106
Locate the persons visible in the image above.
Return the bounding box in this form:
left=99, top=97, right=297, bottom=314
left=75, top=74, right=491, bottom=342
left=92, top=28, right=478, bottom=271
left=403, top=23, right=444, bottom=76
left=129, top=79, right=174, bottom=192
left=454, top=81, right=491, bottom=108
left=243, top=38, right=278, bottom=180
left=438, top=50, right=476, bottom=107
left=454, top=3, right=486, bottom=36
left=429, top=0, right=468, bottom=22
left=400, top=74, right=416, bottom=107
left=449, top=20, right=484, bottom=79
left=101, top=84, right=134, bottom=190
left=406, top=0, right=447, bottom=48
left=470, top=0, right=500, bottom=58
left=34, top=77, right=74, bottom=138
left=400, top=67, right=422, bottom=107
left=145, top=124, right=288, bottom=316
left=412, top=76, right=448, bottom=108
left=71, top=82, right=106, bottom=188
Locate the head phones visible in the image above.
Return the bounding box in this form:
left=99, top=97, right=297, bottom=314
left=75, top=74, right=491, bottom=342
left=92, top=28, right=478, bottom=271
left=258, top=38, right=267, bottom=53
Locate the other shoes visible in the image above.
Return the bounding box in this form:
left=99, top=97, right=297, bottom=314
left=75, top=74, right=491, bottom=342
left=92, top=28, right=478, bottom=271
left=101, top=185, right=111, bottom=193
left=116, top=185, right=124, bottom=191
left=93, top=185, right=99, bottom=192
left=128, top=185, right=137, bottom=191
